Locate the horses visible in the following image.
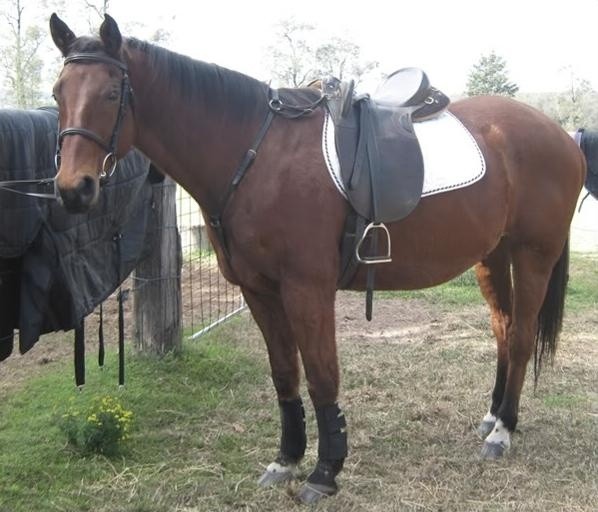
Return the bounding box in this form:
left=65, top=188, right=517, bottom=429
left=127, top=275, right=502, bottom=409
left=49, top=12, right=587, bottom=508
